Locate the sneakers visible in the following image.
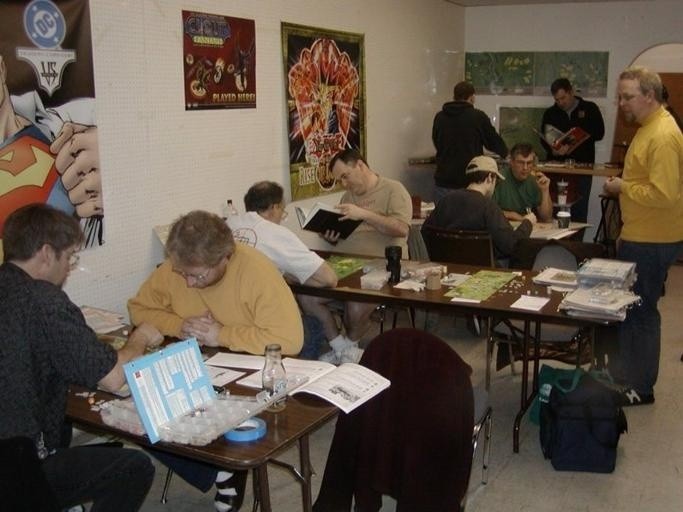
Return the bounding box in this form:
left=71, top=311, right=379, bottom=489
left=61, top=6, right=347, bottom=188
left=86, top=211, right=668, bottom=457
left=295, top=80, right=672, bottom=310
left=316, top=339, right=365, bottom=366
left=618, top=387, right=656, bottom=408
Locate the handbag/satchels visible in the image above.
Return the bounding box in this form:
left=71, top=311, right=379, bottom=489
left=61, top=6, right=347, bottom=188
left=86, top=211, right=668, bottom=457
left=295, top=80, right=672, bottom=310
left=528, top=363, right=614, bottom=428
left=538, top=373, right=631, bottom=475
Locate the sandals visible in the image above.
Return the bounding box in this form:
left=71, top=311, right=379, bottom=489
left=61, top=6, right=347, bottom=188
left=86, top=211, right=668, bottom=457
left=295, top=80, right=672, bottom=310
left=213, top=466, right=250, bottom=512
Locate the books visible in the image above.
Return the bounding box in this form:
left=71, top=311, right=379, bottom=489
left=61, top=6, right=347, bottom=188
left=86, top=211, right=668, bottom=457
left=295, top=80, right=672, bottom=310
left=294, top=201, right=364, bottom=242
left=533, top=122, right=590, bottom=157
left=237, top=355, right=389, bottom=416
left=562, top=255, right=639, bottom=323
left=533, top=265, right=576, bottom=289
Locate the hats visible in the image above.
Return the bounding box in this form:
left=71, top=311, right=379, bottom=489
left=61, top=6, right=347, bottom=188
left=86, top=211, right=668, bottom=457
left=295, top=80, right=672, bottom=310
left=465, top=155, right=507, bottom=182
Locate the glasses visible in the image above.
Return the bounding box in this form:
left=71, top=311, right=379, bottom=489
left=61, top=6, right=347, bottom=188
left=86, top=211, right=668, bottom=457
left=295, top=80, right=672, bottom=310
left=170, top=265, right=211, bottom=282
left=63, top=248, right=81, bottom=271
left=279, top=207, right=289, bottom=220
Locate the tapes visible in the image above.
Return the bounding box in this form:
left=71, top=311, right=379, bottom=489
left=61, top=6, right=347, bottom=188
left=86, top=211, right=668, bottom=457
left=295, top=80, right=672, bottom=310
left=224, top=417, right=267, bottom=443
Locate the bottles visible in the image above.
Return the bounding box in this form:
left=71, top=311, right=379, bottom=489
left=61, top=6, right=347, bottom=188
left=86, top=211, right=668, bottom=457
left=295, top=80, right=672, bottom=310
left=259, top=343, right=288, bottom=414
left=221, top=199, right=238, bottom=219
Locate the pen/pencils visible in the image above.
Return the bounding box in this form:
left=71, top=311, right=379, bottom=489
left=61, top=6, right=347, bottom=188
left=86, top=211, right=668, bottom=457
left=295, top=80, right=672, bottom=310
left=526, top=207, right=531, bottom=215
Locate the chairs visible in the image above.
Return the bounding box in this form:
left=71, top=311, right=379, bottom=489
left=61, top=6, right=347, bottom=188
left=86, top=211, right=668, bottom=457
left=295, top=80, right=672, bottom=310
left=359, top=328, right=493, bottom=512
left=160, top=312, right=322, bottom=512
left=326, top=224, right=422, bottom=334
left=420, top=226, right=494, bottom=337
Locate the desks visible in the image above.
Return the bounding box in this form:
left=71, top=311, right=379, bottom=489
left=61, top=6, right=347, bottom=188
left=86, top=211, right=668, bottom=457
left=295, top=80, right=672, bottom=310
left=284, top=248, right=615, bottom=453
left=409, top=200, right=585, bottom=270
left=64, top=322, right=343, bottom=512
left=408, top=156, right=623, bottom=219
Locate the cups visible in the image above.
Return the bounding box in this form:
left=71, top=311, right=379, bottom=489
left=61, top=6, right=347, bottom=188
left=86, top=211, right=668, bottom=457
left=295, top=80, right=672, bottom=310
left=563, top=158, right=574, bottom=168
left=384, top=245, right=401, bottom=283
left=426, top=275, right=442, bottom=292
left=555, top=210, right=571, bottom=230
left=556, top=181, right=568, bottom=206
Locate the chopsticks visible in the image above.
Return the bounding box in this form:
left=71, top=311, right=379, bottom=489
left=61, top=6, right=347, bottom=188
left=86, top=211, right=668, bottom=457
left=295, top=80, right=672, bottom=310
left=524, top=207, right=533, bottom=216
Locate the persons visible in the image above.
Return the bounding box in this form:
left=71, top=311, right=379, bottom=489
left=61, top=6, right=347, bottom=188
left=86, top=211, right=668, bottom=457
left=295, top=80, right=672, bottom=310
left=432, top=80, right=506, bottom=208
left=0, top=55, right=104, bottom=254
left=603, top=67, right=681, bottom=407
left=420, top=155, right=538, bottom=338
left=0, top=202, right=164, bottom=511
left=300, top=152, right=412, bottom=364
left=541, top=77, right=606, bottom=242
left=492, top=141, right=553, bottom=222
left=126, top=210, right=307, bottom=512
left=658, top=86, right=680, bottom=297
left=225, top=182, right=337, bottom=361
